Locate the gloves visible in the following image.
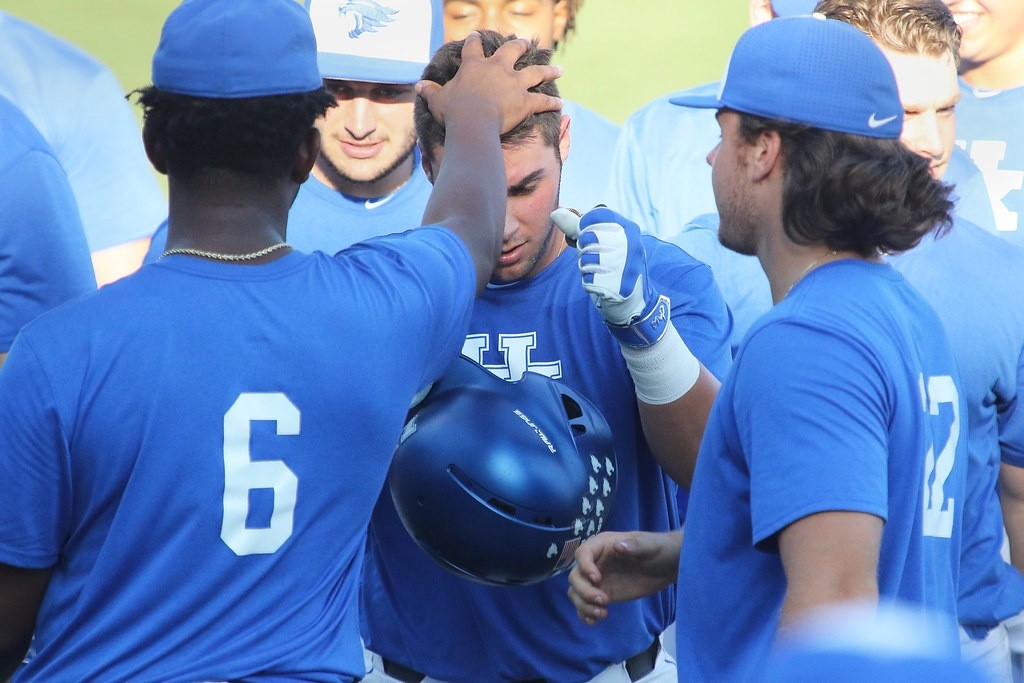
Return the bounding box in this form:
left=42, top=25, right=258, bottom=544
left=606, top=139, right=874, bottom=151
left=549, top=206, right=671, bottom=349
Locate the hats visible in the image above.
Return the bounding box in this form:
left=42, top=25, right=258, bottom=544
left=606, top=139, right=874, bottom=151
left=669, top=16, right=902, bottom=137
left=309, top=0, right=444, bottom=85
left=769, top=0, right=821, bottom=17
left=151, top=0, right=322, bottom=98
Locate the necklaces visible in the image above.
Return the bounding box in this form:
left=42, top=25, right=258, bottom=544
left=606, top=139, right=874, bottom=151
left=782, top=252, right=839, bottom=304
left=159, top=242, right=294, bottom=261
left=559, top=232, right=566, bottom=259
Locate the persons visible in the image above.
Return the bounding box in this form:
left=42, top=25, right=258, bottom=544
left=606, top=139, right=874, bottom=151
left=0, top=0, right=1024, bottom=683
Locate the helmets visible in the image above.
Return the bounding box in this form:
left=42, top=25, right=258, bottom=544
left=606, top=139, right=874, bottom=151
left=389, top=356, right=620, bottom=587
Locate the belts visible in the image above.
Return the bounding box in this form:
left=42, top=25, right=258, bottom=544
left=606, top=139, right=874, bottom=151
left=384, top=639, right=660, bottom=683
